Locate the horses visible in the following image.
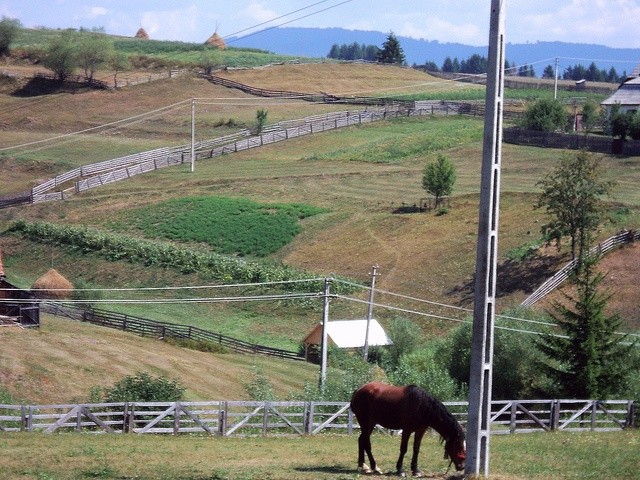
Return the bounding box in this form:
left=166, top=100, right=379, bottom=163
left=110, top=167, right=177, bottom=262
left=350, top=383, right=466, bottom=477
